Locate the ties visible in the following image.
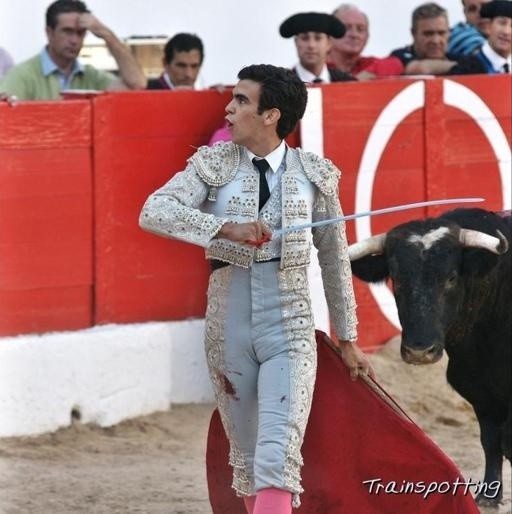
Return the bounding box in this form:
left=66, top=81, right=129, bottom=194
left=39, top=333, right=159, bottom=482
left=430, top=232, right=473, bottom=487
left=253, top=158, right=271, bottom=212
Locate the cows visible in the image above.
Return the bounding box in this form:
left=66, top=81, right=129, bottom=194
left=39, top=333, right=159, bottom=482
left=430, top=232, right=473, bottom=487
left=346, top=207, right=512, bottom=514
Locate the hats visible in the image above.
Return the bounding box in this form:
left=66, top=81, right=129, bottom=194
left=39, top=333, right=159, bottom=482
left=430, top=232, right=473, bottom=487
left=280, top=12, right=345, bottom=38
left=480, top=1, right=512, bottom=18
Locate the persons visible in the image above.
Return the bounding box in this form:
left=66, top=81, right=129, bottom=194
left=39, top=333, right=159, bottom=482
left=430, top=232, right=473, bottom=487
left=136, top=62, right=377, bottom=514
left=2, top=0, right=512, bottom=105
